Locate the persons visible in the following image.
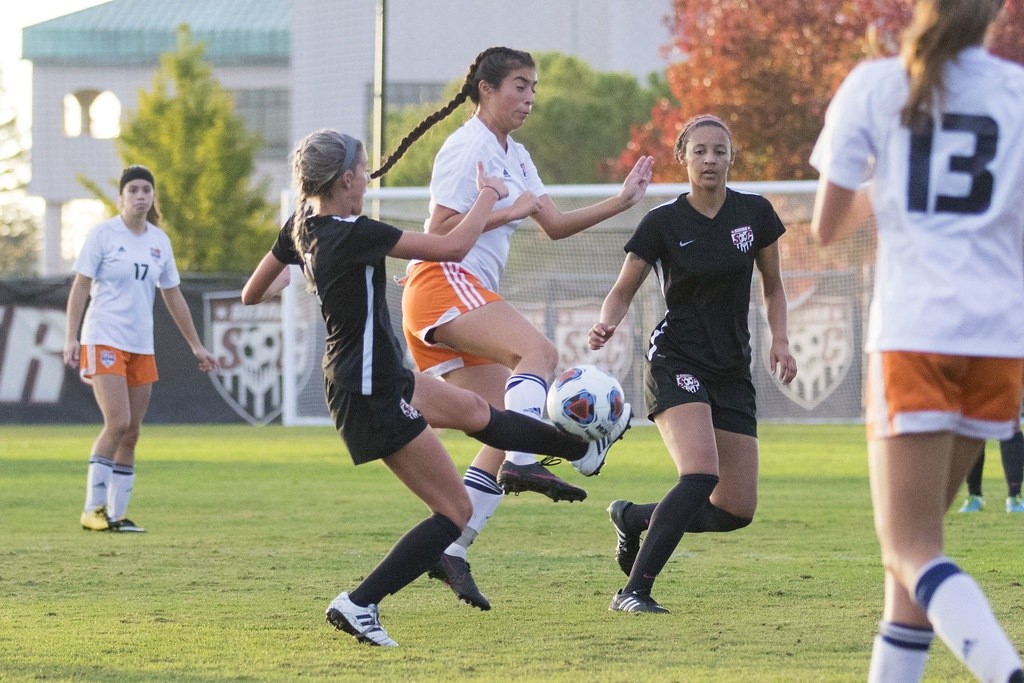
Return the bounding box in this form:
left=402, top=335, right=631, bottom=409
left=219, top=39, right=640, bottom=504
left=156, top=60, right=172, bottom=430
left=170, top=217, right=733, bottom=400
left=588, top=114, right=797, bottom=613
left=64, top=165, right=221, bottom=533
left=371, top=46, right=654, bottom=612
left=808, top=1, right=1024, bottom=683
left=958, top=430, right=1023, bottom=513
left=242, top=128, right=635, bottom=648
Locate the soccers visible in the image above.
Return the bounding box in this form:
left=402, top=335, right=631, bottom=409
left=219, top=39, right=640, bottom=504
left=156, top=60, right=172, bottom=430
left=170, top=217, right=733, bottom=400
left=546, top=363, right=626, bottom=441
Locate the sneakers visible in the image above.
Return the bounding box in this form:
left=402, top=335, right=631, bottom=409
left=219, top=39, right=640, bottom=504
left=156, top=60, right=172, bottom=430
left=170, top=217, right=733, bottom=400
left=497, top=454, right=587, bottom=503
left=568, top=402, right=634, bottom=477
left=326, top=592, right=399, bottom=646
left=427, top=553, right=491, bottom=611
left=80, top=505, right=117, bottom=533
left=607, top=588, right=670, bottom=614
left=109, top=519, right=146, bottom=532
left=958, top=496, right=983, bottom=512
left=606, top=499, right=643, bottom=577
left=1006, top=497, right=1024, bottom=513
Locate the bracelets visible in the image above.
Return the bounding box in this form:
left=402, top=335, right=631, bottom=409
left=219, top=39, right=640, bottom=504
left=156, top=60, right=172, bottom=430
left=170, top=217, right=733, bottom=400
left=481, top=185, right=500, bottom=200
